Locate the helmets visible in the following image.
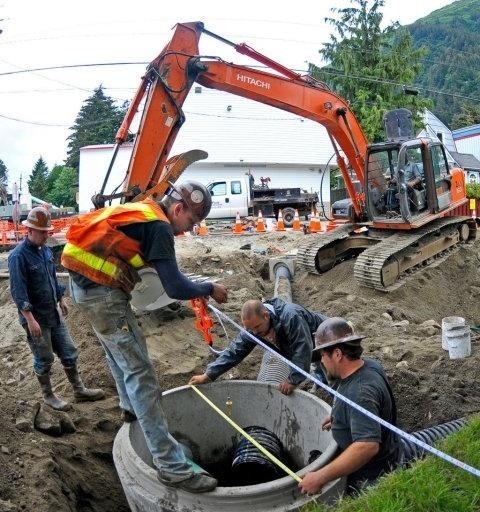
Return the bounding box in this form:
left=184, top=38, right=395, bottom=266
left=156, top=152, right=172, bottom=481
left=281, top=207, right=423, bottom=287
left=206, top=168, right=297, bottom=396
left=22, top=207, right=55, bottom=231
left=311, top=317, right=366, bottom=352
left=167, top=181, right=212, bottom=228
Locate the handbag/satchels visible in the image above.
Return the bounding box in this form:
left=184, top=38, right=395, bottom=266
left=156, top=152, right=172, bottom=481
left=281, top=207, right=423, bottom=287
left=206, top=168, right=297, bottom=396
left=380, top=446, right=399, bottom=474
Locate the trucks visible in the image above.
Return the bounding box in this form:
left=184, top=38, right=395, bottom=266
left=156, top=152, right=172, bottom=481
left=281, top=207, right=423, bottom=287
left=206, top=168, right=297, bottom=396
left=204, top=174, right=320, bottom=228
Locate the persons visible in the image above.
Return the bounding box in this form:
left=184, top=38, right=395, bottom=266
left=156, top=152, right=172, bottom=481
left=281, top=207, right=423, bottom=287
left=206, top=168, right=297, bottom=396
left=188, top=297, right=337, bottom=395
left=298, top=315, right=398, bottom=500
left=7, top=206, right=106, bottom=412
left=382, top=151, right=422, bottom=219
left=61, top=180, right=230, bottom=492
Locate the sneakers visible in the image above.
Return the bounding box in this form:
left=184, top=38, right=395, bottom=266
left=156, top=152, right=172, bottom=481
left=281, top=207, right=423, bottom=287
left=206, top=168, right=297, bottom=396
left=156, top=472, right=218, bottom=493
left=121, top=409, right=137, bottom=422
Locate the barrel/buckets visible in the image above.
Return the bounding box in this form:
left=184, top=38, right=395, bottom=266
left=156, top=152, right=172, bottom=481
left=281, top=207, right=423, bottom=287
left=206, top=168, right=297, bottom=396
left=267, top=218, right=276, bottom=231
left=441, top=315, right=465, bottom=352
left=447, top=328, right=471, bottom=359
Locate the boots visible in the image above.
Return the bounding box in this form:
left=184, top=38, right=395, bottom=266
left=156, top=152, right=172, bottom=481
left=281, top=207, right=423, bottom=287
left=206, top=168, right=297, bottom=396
left=35, top=370, right=70, bottom=411
left=63, top=366, right=105, bottom=400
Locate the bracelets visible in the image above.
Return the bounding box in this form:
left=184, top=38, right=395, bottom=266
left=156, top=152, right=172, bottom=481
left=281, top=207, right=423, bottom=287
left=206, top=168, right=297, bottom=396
left=412, top=181, right=415, bottom=185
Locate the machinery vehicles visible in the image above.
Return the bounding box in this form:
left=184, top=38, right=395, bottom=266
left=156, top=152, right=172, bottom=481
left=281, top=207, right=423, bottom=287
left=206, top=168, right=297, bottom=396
left=90, top=21, right=477, bottom=293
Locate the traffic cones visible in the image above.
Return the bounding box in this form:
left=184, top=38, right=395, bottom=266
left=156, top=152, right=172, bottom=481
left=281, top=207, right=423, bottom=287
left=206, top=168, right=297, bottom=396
left=307, top=209, right=322, bottom=232
left=197, top=218, right=210, bottom=236
left=274, top=209, right=286, bottom=231
left=176, top=231, right=186, bottom=237
left=232, top=212, right=245, bottom=234
left=291, top=209, right=301, bottom=232
left=0, top=226, right=10, bottom=246
left=255, top=210, right=267, bottom=233
